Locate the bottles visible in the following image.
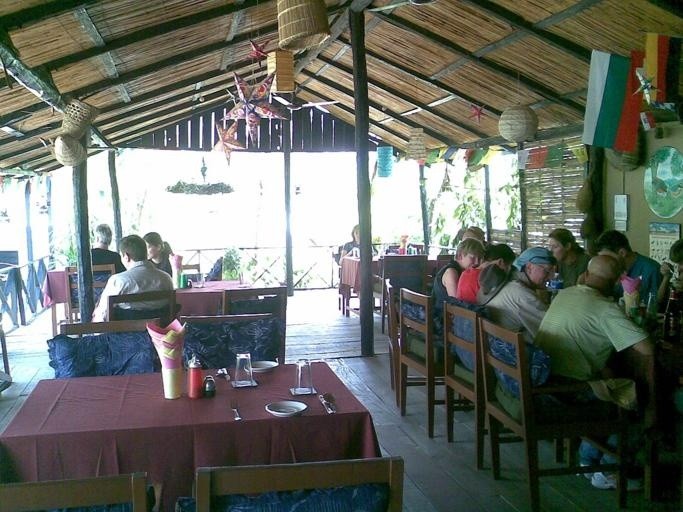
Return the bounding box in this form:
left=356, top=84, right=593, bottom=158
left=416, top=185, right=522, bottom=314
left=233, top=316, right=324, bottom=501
left=629, top=299, right=640, bottom=328
left=186, top=352, right=203, bottom=400
left=663, top=285, right=681, bottom=344
left=179, top=273, right=192, bottom=289
left=643, top=291, right=660, bottom=352
left=551, top=272, right=564, bottom=298
left=203, top=374, right=215, bottom=392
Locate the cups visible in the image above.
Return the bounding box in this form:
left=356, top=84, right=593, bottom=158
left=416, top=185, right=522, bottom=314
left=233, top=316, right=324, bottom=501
left=295, top=360, right=313, bottom=397
left=234, top=352, right=253, bottom=388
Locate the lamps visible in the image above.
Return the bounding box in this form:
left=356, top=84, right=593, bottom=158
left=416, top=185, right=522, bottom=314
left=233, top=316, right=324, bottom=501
left=376, top=105, right=394, bottom=177
left=406, top=76, right=426, bottom=159
left=276, top=0, right=331, bottom=52
left=498, top=40, right=539, bottom=151
left=265, top=38, right=295, bottom=93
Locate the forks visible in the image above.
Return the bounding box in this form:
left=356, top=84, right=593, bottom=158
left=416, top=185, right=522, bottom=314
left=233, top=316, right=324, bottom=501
left=230, top=398, right=242, bottom=420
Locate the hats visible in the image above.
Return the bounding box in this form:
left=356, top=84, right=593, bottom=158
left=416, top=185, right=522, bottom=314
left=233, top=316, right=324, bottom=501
left=474, top=264, right=507, bottom=306
left=512, top=247, right=558, bottom=272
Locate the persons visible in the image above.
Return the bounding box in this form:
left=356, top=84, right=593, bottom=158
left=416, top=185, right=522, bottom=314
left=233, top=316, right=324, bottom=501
left=86, top=222, right=125, bottom=274
left=87, top=234, right=176, bottom=328
left=429, top=225, right=682, bottom=489
left=338, top=223, right=378, bottom=269
left=142, top=231, right=179, bottom=292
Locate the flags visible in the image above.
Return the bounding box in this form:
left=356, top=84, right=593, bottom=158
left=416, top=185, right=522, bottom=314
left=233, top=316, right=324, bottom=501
left=580, top=49, right=644, bottom=152
left=512, top=150, right=528, bottom=168
left=629, top=48, right=649, bottom=100
left=567, top=138, right=590, bottom=164
left=416, top=142, right=502, bottom=165
left=542, top=145, right=564, bottom=166
left=525, top=147, right=549, bottom=169
left=642, top=30, right=683, bottom=102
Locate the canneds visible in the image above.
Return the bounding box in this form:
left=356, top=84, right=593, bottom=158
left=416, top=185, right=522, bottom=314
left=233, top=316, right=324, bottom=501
left=179, top=273, right=188, bottom=289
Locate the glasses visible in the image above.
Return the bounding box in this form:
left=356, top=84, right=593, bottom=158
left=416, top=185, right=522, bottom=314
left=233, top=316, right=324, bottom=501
left=529, top=255, right=556, bottom=263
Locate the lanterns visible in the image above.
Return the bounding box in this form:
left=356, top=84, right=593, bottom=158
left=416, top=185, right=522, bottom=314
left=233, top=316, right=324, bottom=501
left=375, top=145, right=394, bottom=178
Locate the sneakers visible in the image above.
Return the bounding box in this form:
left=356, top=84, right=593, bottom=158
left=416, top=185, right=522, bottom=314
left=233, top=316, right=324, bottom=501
left=580, top=464, right=641, bottom=491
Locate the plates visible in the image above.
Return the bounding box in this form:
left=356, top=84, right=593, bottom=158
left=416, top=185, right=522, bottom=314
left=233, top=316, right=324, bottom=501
left=266, top=399, right=309, bottom=418
left=245, top=360, right=280, bottom=374
